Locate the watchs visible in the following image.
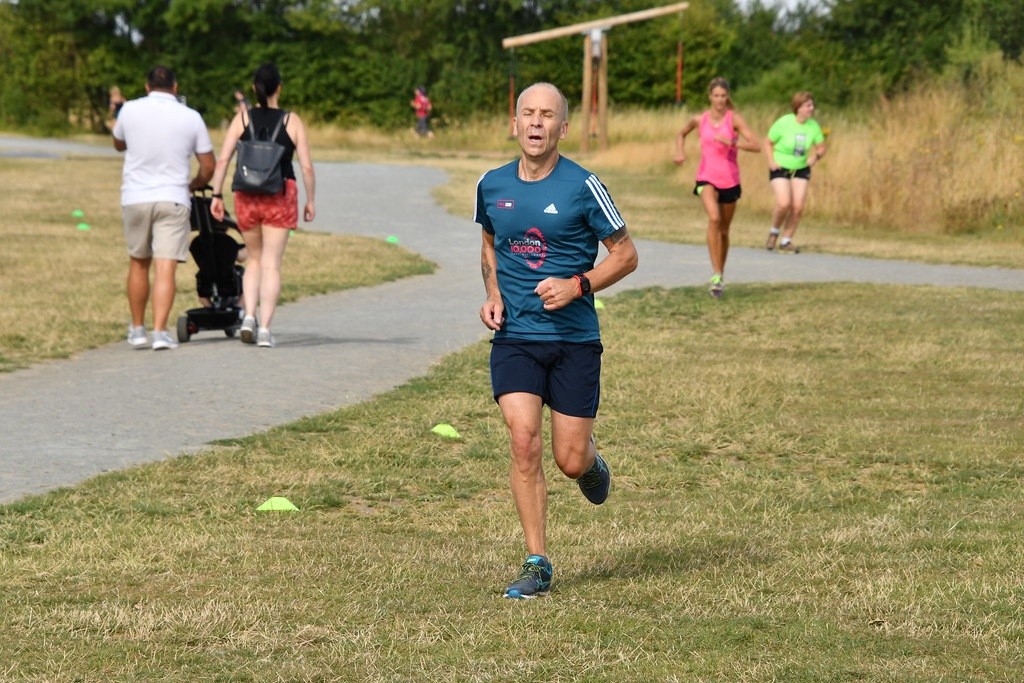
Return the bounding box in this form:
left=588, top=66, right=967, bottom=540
left=573, top=273, right=591, bottom=297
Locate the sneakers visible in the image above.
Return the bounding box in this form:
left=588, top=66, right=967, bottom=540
left=257, top=328, right=277, bottom=347
left=575, top=432, right=611, bottom=506
left=150, top=329, right=177, bottom=349
left=128, top=322, right=148, bottom=345
left=502, top=556, right=552, bottom=600
left=240, top=315, right=260, bottom=343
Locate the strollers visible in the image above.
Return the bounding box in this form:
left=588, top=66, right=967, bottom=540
left=176, top=183, right=259, bottom=343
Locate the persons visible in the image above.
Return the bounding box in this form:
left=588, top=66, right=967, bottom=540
left=673, top=77, right=761, bottom=298
left=113, top=64, right=217, bottom=349
left=207, top=61, right=316, bottom=350
left=764, top=90, right=826, bottom=253
left=410, top=85, right=433, bottom=139
left=472, top=83, right=639, bottom=599
left=233, top=88, right=250, bottom=111
left=107, top=85, right=128, bottom=120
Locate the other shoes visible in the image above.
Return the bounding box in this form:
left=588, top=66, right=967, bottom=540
left=709, top=275, right=723, bottom=297
left=779, top=240, right=800, bottom=255
left=767, top=233, right=779, bottom=250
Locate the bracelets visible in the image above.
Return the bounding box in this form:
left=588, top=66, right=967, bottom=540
left=213, top=193, right=222, bottom=198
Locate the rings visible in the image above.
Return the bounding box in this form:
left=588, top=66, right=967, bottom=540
left=547, top=300, right=550, bottom=304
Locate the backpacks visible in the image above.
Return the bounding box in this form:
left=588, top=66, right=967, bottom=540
left=231, top=109, right=286, bottom=193
